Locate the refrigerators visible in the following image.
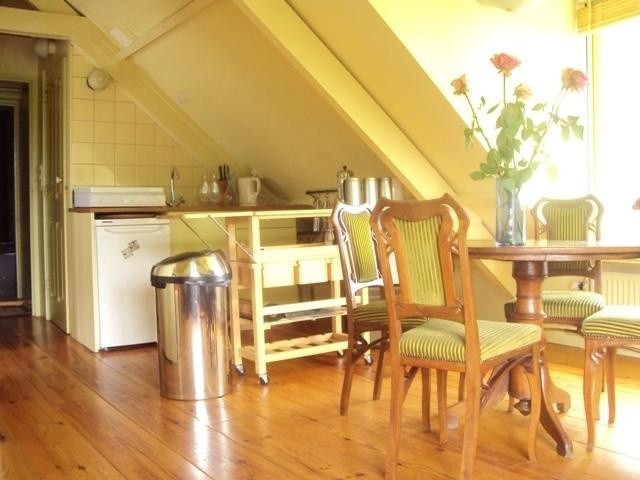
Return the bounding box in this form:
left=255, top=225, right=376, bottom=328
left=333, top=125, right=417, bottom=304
left=95, top=218, right=170, bottom=352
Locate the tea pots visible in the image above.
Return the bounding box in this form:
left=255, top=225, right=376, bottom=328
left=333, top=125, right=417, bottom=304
left=238, top=176, right=262, bottom=207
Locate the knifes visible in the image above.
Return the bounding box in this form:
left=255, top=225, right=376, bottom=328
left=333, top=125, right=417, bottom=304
left=219, top=164, right=231, bottom=181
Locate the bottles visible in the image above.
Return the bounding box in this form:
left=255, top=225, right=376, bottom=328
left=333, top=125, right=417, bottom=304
left=199, top=172, right=220, bottom=207
left=336, top=164, right=354, bottom=206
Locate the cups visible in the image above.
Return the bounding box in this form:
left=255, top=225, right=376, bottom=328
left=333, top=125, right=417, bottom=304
left=344, top=176, right=393, bottom=207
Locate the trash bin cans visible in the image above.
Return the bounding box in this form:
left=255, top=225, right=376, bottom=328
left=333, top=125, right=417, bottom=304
left=151, top=249, right=232, bottom=400
left=0, top=241, right=16, bottom=297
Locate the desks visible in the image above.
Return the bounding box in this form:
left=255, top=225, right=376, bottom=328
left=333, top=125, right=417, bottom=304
left=160, top=207, right=374, bottom=388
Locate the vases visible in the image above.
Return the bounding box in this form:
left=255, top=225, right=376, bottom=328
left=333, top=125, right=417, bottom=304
left=494, top=178, right=527, bottom=246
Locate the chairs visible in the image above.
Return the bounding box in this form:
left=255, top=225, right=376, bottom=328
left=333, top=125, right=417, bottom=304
left=504, top=195, right=603, bottom=420
left=582, top=303, right=640, bottom=452
left=332, top=202, right=467, bottom=433
left=370, top=194, right=544, bottom=478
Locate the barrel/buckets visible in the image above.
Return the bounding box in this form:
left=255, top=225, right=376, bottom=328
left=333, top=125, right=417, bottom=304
left=341, top=176, right=405, bottom=217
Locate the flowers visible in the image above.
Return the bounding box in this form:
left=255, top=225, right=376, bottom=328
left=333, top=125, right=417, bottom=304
left=450, top=52, right=586, bottom=241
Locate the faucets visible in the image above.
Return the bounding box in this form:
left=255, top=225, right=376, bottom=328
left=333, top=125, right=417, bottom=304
left=169, top=167, right=179, bottom=206
